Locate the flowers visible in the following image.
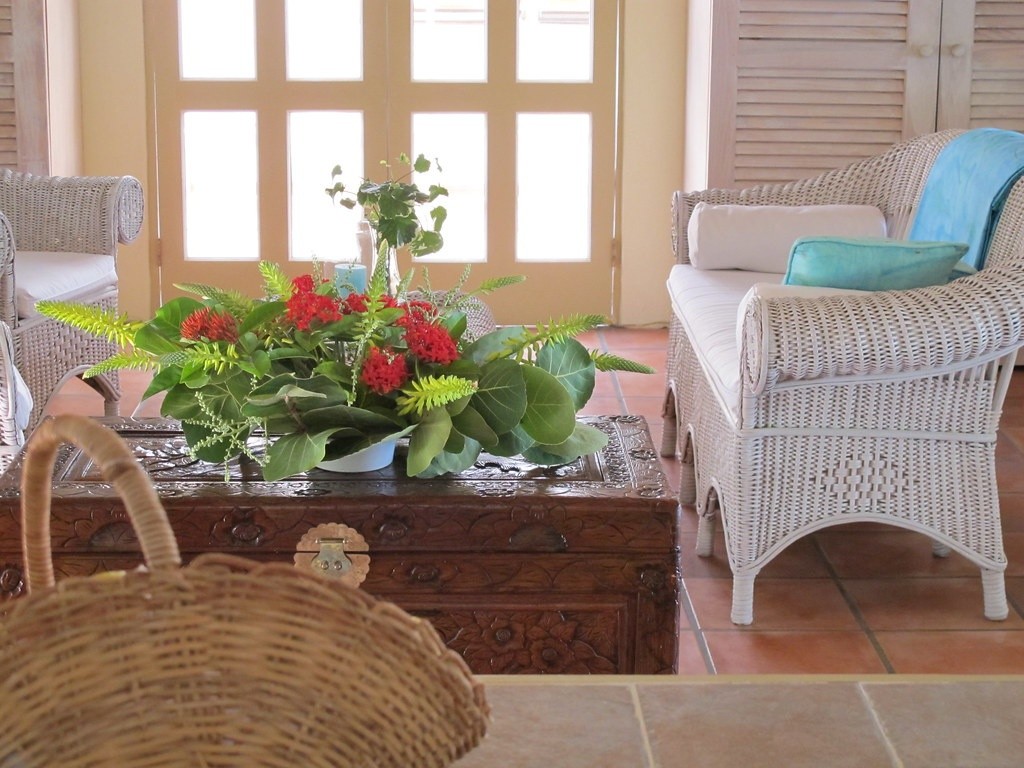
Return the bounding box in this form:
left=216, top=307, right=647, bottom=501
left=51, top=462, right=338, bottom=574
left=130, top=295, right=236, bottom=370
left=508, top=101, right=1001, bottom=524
left=29, top=239, right=657, bottom=478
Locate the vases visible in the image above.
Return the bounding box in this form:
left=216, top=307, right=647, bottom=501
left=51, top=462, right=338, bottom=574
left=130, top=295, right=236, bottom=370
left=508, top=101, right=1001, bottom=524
left=316, top=437, right=396, bottom=474
left=366, top=220, right=409, bottom=304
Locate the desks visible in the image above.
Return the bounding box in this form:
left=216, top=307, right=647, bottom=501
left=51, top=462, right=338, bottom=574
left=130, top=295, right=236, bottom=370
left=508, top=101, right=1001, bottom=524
left=454, top=669, right=1024, bottom=768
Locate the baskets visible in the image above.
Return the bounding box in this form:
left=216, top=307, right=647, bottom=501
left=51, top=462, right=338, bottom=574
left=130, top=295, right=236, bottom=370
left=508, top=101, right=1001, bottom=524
left=0, top=414, right=492, bottom=768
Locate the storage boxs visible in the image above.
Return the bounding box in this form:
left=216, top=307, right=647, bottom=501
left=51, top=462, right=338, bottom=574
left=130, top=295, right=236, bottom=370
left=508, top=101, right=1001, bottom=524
left=0, top=416, right=683, bottom=674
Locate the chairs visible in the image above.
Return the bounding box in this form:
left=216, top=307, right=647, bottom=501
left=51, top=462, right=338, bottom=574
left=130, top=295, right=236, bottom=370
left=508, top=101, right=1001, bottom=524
left=0, top=166, right=145, bottom=476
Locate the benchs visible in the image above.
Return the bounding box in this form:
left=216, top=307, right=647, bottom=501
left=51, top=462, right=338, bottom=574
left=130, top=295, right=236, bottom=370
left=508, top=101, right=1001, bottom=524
left=661, top=126, right=1024, bottom=626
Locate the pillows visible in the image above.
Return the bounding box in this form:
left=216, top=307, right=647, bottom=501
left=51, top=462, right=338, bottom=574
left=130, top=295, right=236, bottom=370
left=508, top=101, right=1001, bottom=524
left=786, top=233, right=971, bottom=291
left=685, top=203, right=889, bottom=274
